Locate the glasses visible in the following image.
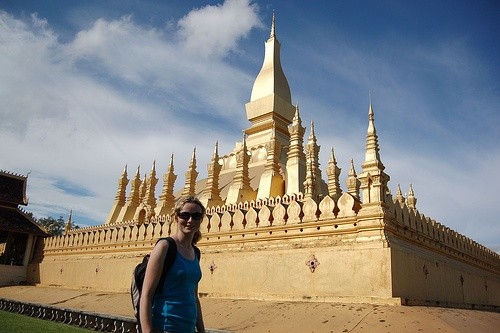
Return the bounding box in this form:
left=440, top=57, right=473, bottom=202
left=177, top=212, right=202, bottom=220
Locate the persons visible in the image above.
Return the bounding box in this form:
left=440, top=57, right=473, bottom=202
left=139, top=198, right=205, bottom=333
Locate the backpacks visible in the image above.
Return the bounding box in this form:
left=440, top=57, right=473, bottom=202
left=130, top=236, right=200, bottom=319
